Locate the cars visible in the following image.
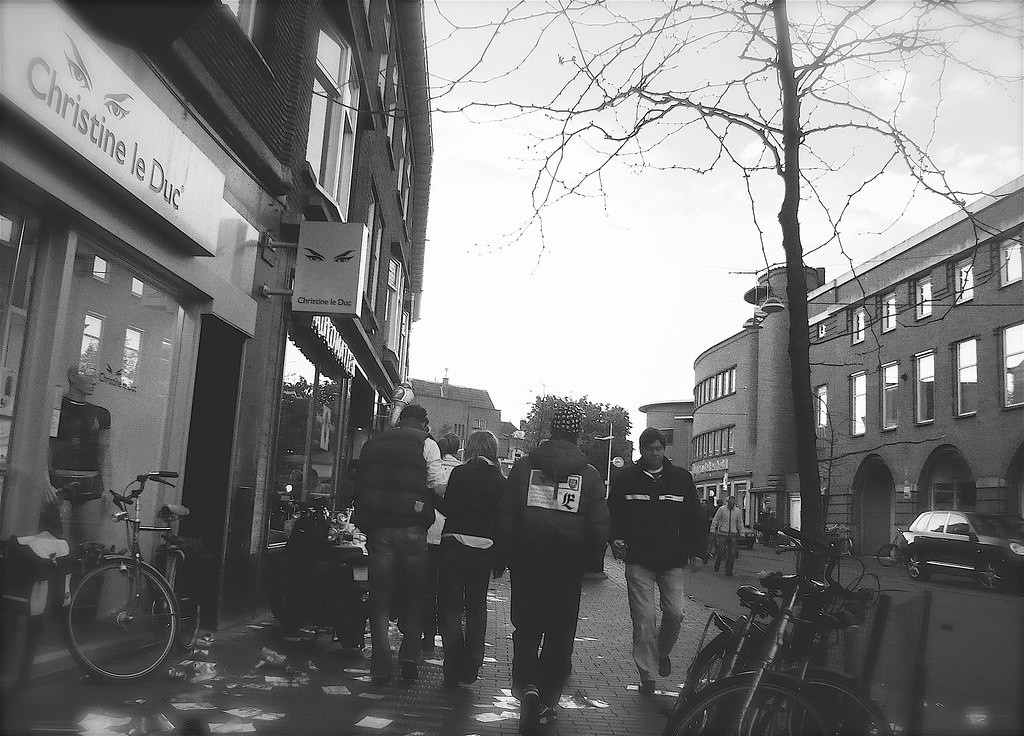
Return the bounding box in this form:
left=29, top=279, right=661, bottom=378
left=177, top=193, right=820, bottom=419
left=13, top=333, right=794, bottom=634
left=737, top=523, right=756, bottom=549
left=898, top=508, right=1024, bottom=593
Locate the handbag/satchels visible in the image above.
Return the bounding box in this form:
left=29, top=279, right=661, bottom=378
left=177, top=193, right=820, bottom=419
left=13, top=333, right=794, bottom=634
left=0, top=531, right=84, bottom=616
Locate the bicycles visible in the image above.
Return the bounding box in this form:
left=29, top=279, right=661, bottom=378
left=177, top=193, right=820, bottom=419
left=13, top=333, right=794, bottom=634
left=661, top=513, right=889, bottom=736
left=876, top=524, right=909, bottom=568
left=63, top=468, right=205, bottom=686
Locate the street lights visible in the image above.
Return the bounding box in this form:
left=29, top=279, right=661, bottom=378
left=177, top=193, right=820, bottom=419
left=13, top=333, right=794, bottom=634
left=597, top=425, right=624, bottom=502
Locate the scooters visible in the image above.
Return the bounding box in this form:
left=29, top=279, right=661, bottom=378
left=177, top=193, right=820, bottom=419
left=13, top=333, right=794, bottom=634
left=271, top=484, right=375, bottom=658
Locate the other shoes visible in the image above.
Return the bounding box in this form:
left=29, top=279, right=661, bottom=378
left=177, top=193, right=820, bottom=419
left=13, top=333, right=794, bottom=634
left=372, top=678, right=391, bottom=693
left=421, top=637, right=433, bottom=651
left=519, top=686, right=541, bottom=736
left=725, top=568, right=733, bottom=576
left=714, top=564, right=719, bottom=572
left=658, top=649, right=670, bottom=677
left=639, top=680, right=655, bottom=695
left=400, top=660, right=418, bottom=680
left=539, top=705, right=557, bottom=718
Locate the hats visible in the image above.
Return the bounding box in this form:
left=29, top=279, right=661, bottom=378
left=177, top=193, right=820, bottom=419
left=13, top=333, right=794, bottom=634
left=552, top=402, right=583, bottom=435
left=400, top=404, right=429, bottom=423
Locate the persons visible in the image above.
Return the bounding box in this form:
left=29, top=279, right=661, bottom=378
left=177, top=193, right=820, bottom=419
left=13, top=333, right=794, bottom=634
left=355, top=405, right=506, bottom=689
left=45, top=360, right=111, bottom=550
left=492, top=402, right=611, bottom=736
left=606, top=426, right=703, bottom=695
left=701, top=495, right=745, bottom=577
left=289, top=468, right=330, bottom=503
